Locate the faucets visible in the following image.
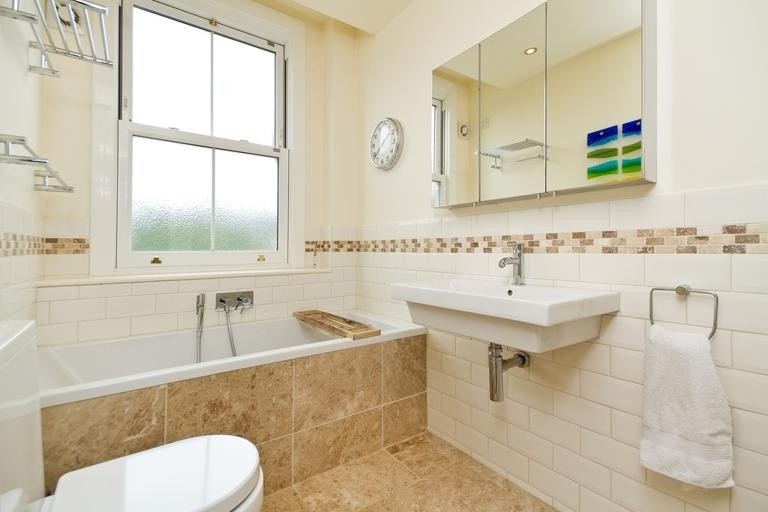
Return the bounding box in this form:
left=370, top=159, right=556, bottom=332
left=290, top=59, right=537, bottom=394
left=499, top=241, right=524, bottom=283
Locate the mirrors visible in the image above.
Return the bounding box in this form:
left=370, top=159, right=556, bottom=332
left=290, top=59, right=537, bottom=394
left=430, top=40, right=480, bottom=209
left=548, top=1, right=659, bottom=196
left=478, top=0, right=547, bottom=205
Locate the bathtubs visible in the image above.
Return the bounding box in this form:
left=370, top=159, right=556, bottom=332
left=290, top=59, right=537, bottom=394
left=40, top=313, right=430, bottom=409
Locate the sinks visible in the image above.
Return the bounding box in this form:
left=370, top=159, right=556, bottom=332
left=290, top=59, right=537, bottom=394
left=390, top=277, right=620, bottom=354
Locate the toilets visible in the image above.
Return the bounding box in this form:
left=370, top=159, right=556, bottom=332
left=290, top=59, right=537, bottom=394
left=0, top=316, right=264, bottom=511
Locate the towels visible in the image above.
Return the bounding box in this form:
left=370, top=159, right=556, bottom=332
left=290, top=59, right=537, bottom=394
left=638, top=322, right=736, bottom=490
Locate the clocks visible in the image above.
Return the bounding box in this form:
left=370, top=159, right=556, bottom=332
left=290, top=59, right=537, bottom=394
left=368, top=116, right=405, bottom=170
